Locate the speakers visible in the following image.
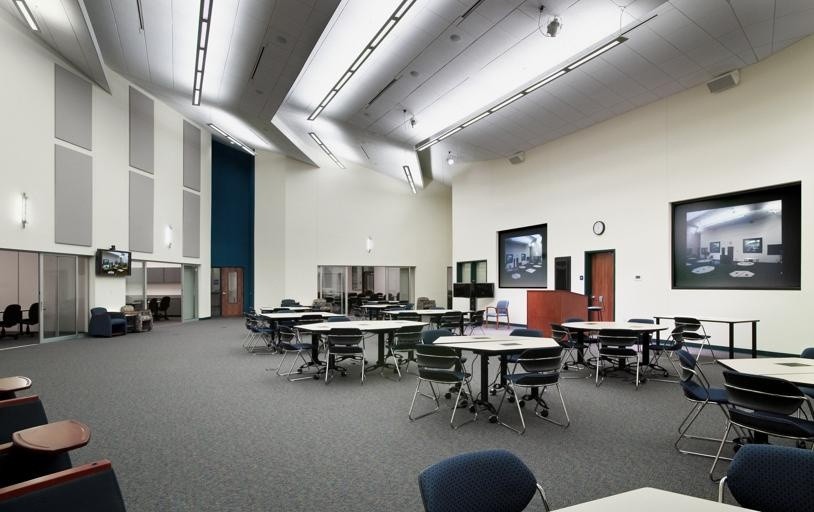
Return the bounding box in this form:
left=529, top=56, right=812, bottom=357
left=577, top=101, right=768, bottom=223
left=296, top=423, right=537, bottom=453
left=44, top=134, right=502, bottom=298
left=704, top=69, right=740, bottom=95
left=507, top=151, right=525, bottom=164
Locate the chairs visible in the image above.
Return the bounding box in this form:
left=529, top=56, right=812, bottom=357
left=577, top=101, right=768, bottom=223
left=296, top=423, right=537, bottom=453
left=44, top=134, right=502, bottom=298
left=0, top=375, right=126, bottom=511
left=1, top=304, right=23, bottom=340
left=88, top=296, right=170, bottom=337
left=19, top=302, right=39, bottom=334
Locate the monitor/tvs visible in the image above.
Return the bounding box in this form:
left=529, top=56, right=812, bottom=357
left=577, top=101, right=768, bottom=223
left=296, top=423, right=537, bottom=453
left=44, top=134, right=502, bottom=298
left=498, top=223, right=548, bottom=288
left=96, top=249, right=131, bottom=277
left=672, top=181, right=801, bottom=290
